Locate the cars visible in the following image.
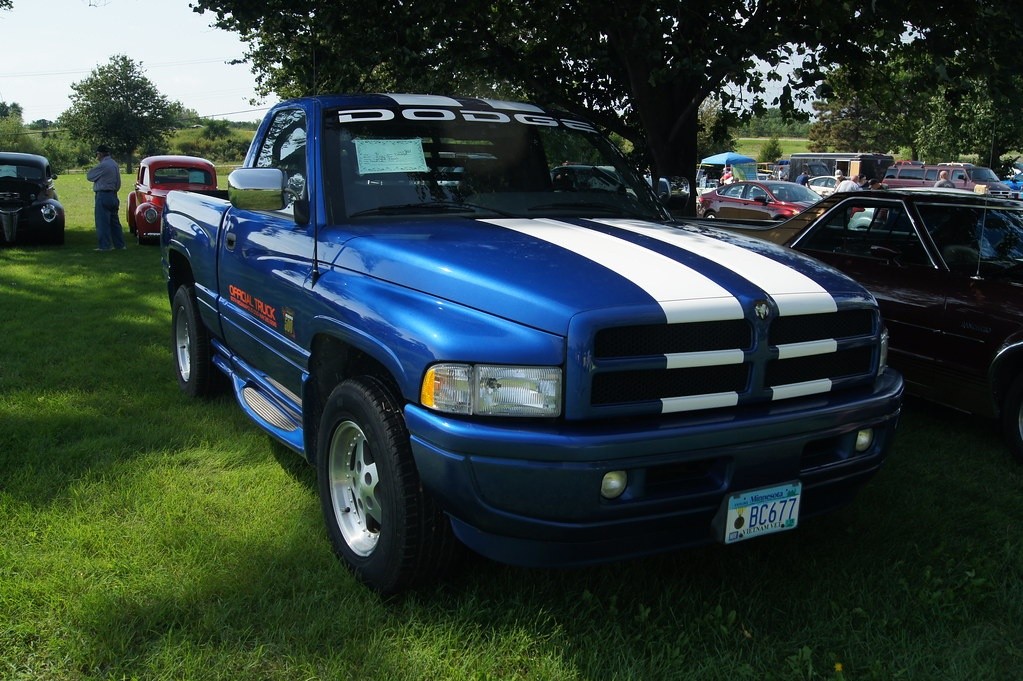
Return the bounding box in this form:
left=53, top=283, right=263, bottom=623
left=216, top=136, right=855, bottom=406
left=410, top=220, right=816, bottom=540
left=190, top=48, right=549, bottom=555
left=698, top=181, right=822, bottom=220
left=805, top=175, right=836, bottom=195
left=0, top=152, right=64, bottom=246
left=999, top=171, right=1023, bottom=192
left=127, top=155, right=217, bottom=244
left=678, top=189, right=1023, bottom=442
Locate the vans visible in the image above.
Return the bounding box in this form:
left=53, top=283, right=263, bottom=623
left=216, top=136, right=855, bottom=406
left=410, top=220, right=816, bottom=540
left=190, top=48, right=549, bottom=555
left=882, top=160, right=1012, bottom=198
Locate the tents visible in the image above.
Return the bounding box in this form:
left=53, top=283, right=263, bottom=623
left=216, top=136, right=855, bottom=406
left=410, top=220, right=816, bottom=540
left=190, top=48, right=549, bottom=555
left=700, top=151, right=756, bottom=189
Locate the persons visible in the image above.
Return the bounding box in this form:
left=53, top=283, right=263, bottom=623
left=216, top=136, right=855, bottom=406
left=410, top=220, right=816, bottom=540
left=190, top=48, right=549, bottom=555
left=779, top=168, right=786, bottom=179
left=832, top=170, right=846, bottom=191
left=858, top=175, right=870, bottom=190
left=834, top=175, right=863, bottom=193
left=720, top=168, right=731, bottom=184
left=85, top=145, right=127, bottom=252
left=868, top=178, right=889, bottom=189
left=933, top=170, right=955, bottom=189
left=1008, top=178, right=1021, bottom=200
left=794, top=171, right=811, bottom=190
left=852, top=175, right=863, bottom=191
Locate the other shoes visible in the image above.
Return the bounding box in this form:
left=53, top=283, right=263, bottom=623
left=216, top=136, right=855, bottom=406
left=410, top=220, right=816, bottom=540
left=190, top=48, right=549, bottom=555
left=94, top=246, right=115, bottom=251
left=114, top=245, right=128, bottom=250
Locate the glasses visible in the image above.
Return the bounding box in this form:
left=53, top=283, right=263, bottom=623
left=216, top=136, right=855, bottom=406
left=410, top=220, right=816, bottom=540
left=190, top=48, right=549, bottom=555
left=95, top=152, right=101, bottom=157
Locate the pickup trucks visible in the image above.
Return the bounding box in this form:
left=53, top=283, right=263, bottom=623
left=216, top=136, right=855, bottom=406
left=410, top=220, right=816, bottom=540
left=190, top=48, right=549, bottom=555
left=164, top=90, right=906, bottom=594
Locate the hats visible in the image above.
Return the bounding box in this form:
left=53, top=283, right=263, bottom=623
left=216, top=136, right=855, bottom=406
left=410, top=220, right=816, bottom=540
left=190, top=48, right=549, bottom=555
left=92, top=144, right=111, bottom=153
